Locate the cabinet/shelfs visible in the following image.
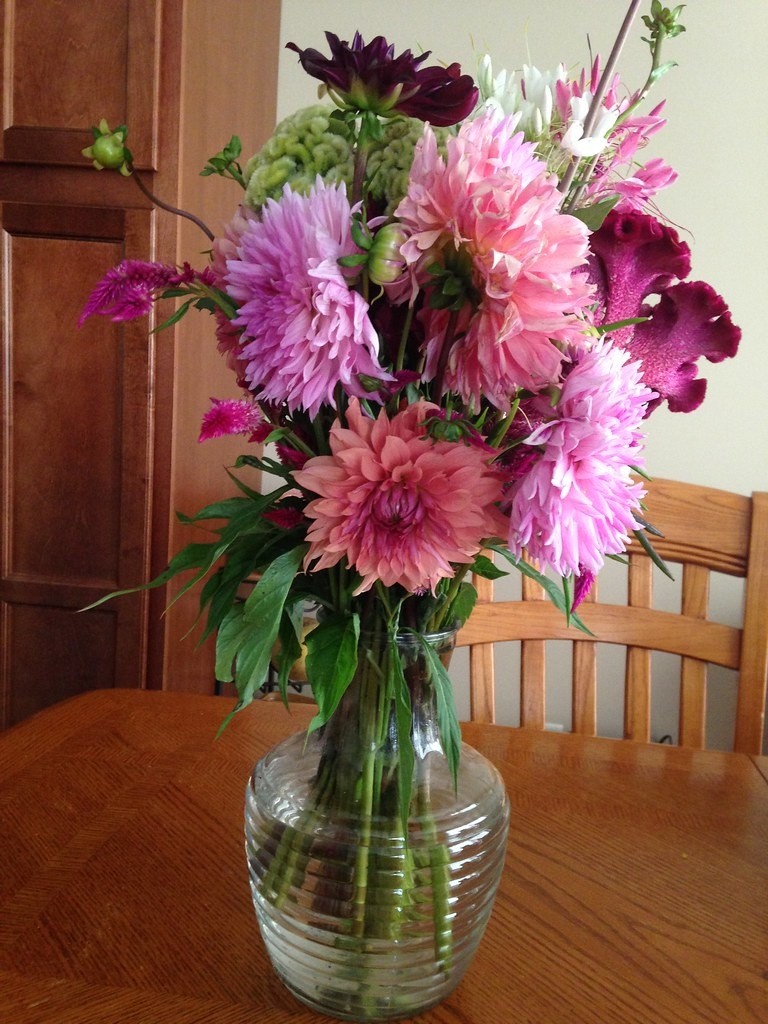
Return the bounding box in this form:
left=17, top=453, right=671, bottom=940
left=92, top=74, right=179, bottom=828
left=0, top=0, right=283, bottom=728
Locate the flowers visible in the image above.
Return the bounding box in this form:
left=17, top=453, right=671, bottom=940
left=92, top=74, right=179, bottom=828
left=73, top=0, right=744, bottom=1024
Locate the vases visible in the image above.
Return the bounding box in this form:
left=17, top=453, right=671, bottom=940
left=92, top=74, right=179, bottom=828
left=240, top=635, right=509, bottom=1022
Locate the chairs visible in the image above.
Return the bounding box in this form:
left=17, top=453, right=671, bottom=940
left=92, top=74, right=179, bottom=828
left=455, top=469, right=768, bottom=757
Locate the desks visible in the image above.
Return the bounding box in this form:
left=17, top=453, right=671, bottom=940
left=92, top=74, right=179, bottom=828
left=1, top=687, right=768, bottom=1024
